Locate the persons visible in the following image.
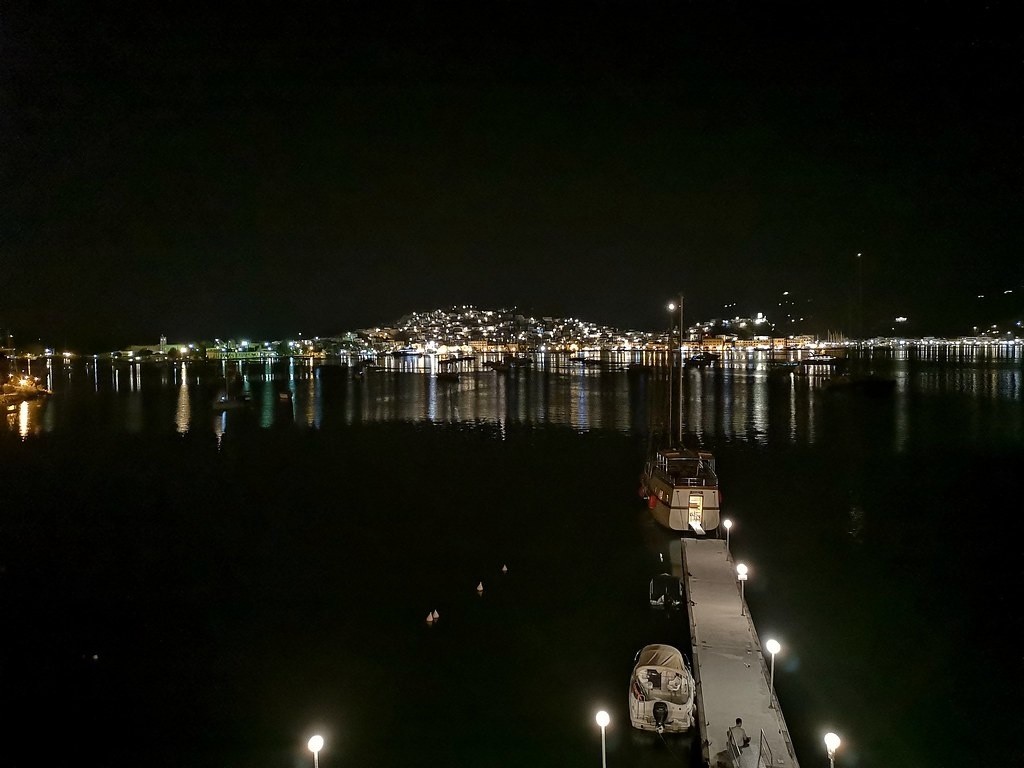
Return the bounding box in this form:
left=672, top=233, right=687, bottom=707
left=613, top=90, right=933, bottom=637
left=727, top=718, right=751, bottom=747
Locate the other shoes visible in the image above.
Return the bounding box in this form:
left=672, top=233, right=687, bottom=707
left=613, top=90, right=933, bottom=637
left=743, top=744, right=749, bottom=747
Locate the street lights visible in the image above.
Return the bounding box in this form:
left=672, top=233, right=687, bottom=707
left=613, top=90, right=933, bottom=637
left=824, top=732, right=841, bottom=768
left=724, top=519, right=734, bottom=561
left=895, top=315, right=908, bottom=340
left=765, top=638, right=780, bottom=709
left=737, top=565, right=748, bottom=616
left=667, top=295, right=684, bottom=441
left=595, top=711, right=610, bottom=768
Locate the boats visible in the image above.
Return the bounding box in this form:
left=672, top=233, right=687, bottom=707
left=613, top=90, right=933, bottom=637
left=483, top=355, right=532, bottom=372
left=629, top=643, right=696, bottom=732
left=436, top=372, right=459, bottom=382
left=685, top=351, right=721, bottom=368
left=641, top=448, right=722, bottom=533
left=649, top=574, right=684, bottom=612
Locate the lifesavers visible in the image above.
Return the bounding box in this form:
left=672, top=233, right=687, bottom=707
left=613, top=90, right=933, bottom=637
left=648, top=495, right=656, bottom=509
left=719, top=493, right=722, bottom=503
